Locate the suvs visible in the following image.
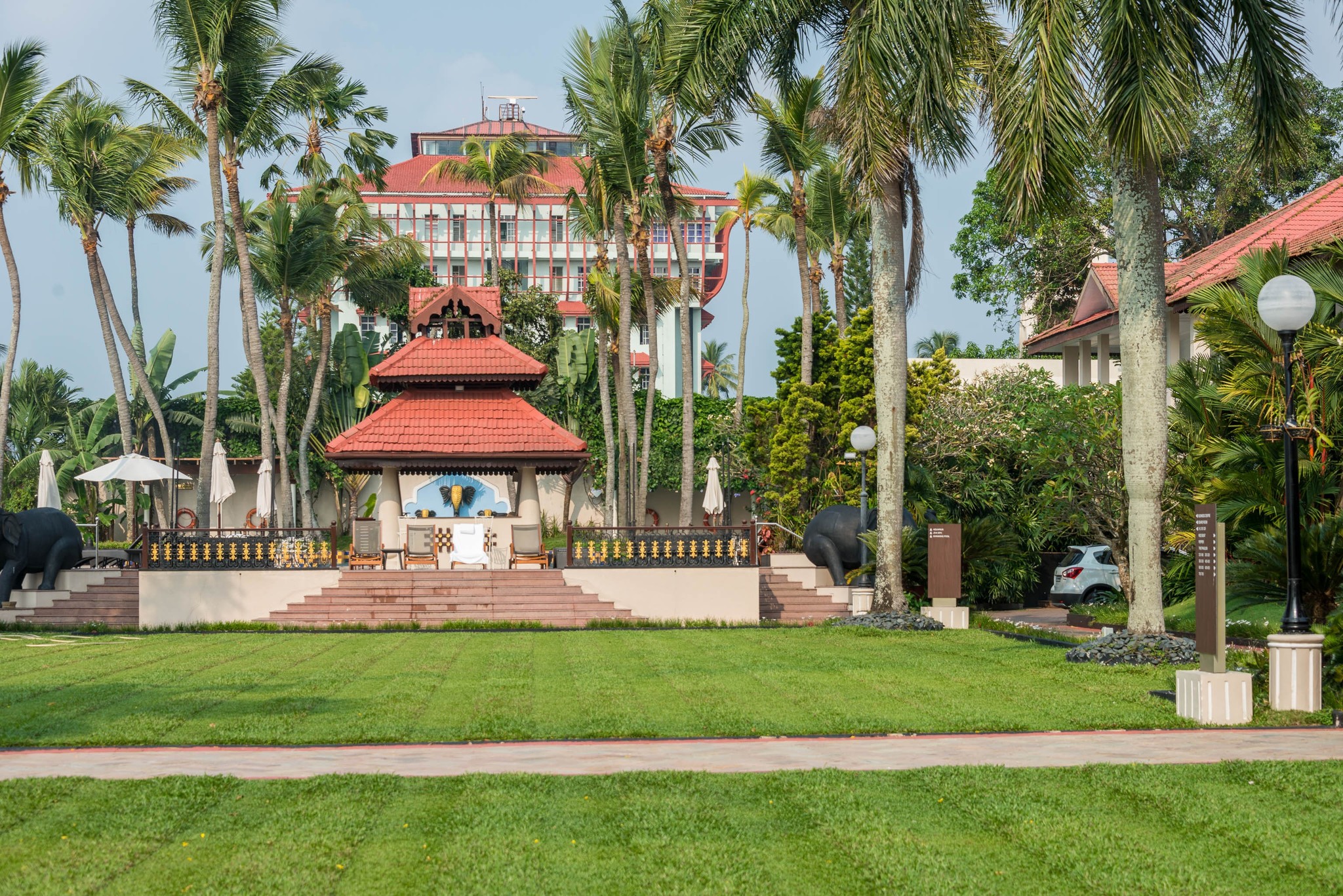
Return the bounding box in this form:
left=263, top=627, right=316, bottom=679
left=1048, top=543, right=1193, bottom=611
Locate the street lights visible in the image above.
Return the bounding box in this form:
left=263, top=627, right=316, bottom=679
left=1258, top=275, right=1328, bottom=714
left=850, top=425, right=876, bottom=619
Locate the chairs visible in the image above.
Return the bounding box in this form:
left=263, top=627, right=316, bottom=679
left=80, top=533, right=143, bottom=569
left=450, top=524, right=489, bottom=569
left=510, top=524, right=548, bottom=570
left=404, top=524, right=439, bottom=570
left=350, top=521, right=383, bottom=570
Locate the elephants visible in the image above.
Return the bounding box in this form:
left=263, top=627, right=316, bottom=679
left=803, top=505, right=939, bottom=586
left=0, top=506, right=85, bottom=608
left=440, top=484, right=476, bottom=517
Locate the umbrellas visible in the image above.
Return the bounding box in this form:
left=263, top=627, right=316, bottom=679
left=210, top=439, right=237, bottom=538
left=38, top=449, right=62, bottom=511
left=74, top=449, right=193, bottom=543
left=257, top=456, right=277, bottom=537
left=702, top=454, right=726, bottom=526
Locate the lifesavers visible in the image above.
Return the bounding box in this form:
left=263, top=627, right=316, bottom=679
left=703, top=510, right=722, bottom=532
left=176, top=508, right=196, bottom=529
left=644, top=508, right=659, bottom=532
left=246, top=508, right=267, bottom=529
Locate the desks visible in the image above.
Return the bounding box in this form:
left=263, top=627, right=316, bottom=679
left=381, top=549, right=404, bottom=570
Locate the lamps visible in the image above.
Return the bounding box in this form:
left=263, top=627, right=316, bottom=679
left=455, top=384, right=465, bottom=392
